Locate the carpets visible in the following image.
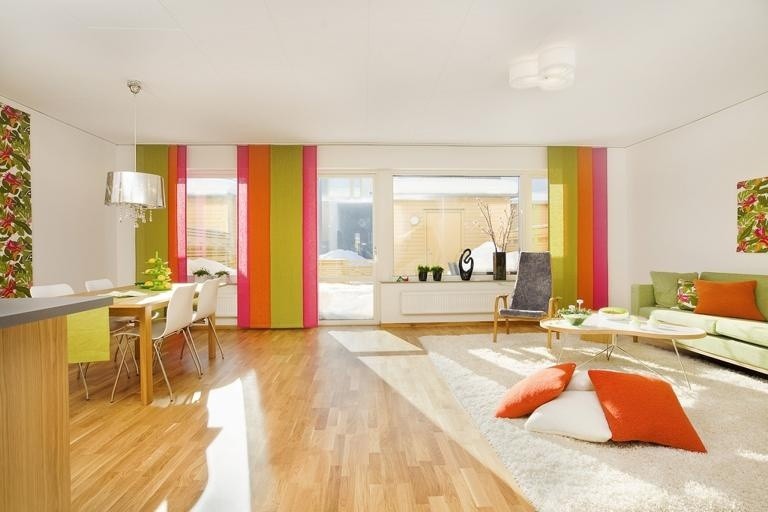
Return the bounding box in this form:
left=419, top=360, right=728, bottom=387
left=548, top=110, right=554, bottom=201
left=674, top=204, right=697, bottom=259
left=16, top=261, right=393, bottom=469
left=415, top=328, right=765, bottom=512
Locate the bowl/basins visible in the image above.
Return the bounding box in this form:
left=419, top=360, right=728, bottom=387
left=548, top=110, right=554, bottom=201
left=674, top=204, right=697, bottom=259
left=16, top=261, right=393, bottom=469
left=600, top=307, right=629, bottom=321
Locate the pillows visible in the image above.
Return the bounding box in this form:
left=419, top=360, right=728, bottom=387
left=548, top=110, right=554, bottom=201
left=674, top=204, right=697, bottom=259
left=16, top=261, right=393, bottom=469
left=648, top=270, right=767, bottom=323
left=492, top=355, right=710, bottom=454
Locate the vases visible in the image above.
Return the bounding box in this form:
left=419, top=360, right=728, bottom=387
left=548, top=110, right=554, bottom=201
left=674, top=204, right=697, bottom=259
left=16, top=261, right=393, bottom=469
left=492, top=252, right=507, bottom=281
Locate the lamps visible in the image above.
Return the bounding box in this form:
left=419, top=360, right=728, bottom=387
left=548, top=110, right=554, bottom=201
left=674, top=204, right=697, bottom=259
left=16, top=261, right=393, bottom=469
left=103, top=78, right=169, bottom=225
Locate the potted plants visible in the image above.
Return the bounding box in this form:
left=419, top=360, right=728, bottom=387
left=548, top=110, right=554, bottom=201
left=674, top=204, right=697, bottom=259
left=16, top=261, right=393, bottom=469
left=194, top=269, right=230, bottom=285
left=417, top=265, right=444, bottom=281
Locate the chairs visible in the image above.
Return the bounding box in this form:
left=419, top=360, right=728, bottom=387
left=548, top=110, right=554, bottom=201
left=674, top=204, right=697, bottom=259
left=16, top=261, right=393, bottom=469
left=28, top=275, right=227, bottom=406
left=487, top=251, right=561, bottom=348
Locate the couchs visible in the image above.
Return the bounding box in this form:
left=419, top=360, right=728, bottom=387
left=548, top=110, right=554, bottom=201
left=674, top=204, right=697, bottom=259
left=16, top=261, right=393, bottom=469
left=623, top=268, right=767, bottom=376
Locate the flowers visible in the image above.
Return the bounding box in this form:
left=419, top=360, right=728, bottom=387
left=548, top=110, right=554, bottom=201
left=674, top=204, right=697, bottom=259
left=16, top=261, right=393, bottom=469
left=142, top=251, right=171, bottom=291
